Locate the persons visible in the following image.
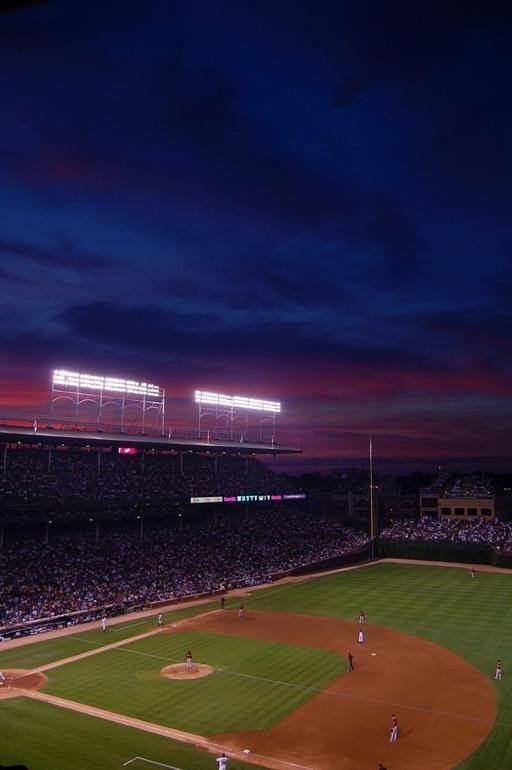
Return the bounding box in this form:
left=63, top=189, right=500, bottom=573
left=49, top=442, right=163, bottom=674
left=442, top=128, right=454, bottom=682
left=215, top=752, right=229, bottom=770
left=378, top=763, right=387, bottom=770
left=389, top=713, right=398, bottom=744
left=470, top=567, right=474, bottom=577
left=185, top=649, right=194, bottom=670
left=371, top=474, right=511, bottom=553
left=494, top=659, right=502, bottom=682
left=346, top=652, right=354, bottom=674
left=1, top=426, right=371, bottom=639
left=357, top=629, right=364, bottom=645
